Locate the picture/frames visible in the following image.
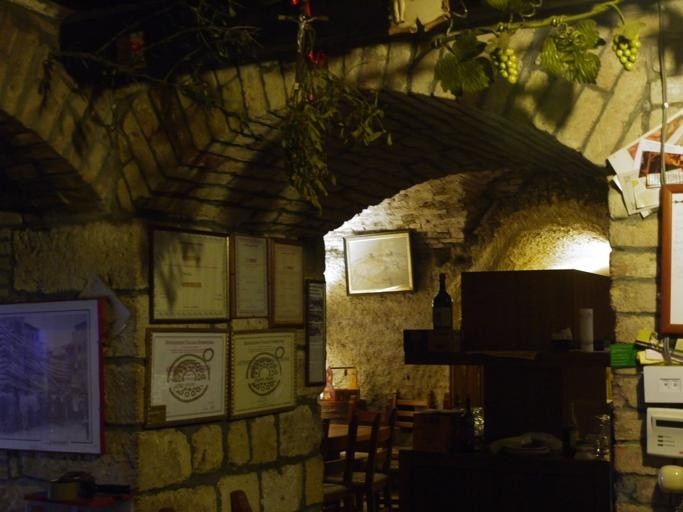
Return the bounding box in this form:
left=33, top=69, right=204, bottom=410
left=0, top=298, right=106, bottom=457
left=342, top=231, right=415, bottom=296
left=146, top=223, right=327, bottom=429
left=660, top=184, right=683, bottom=337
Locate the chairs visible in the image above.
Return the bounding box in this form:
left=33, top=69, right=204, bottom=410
left=322, top=391, right=435, bottom=512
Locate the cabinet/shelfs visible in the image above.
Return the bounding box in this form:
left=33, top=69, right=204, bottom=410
left=400, top=269, right=614, bottom=512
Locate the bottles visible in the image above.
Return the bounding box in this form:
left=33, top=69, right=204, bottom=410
left=432, top=272, right=453, bottom=337
